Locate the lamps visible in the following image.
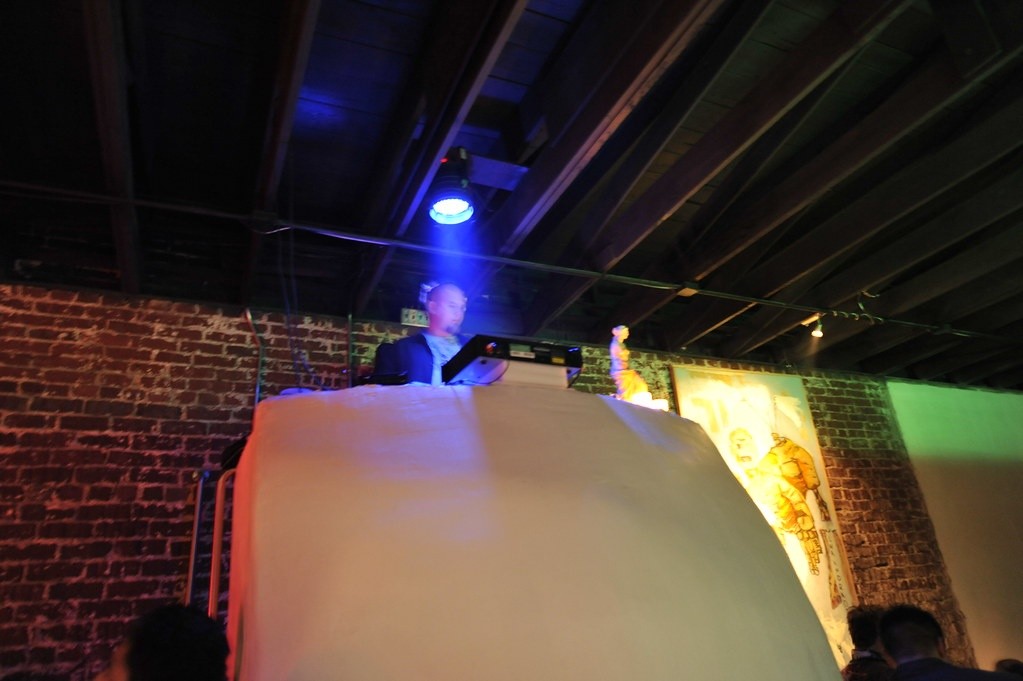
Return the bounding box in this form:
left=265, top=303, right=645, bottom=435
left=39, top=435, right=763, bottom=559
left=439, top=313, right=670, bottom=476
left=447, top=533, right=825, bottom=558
left=812, top=317, right=823, bottom=339
left=427, top=166, right=476, bottom=229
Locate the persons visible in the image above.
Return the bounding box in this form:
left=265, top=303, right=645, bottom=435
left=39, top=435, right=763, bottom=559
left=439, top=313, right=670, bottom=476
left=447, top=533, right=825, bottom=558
left=842, top=604, right=1023, bottom=681
left=111, top=604, right=229, bottom=681
left=373, top=281, right=491, bottom=386
